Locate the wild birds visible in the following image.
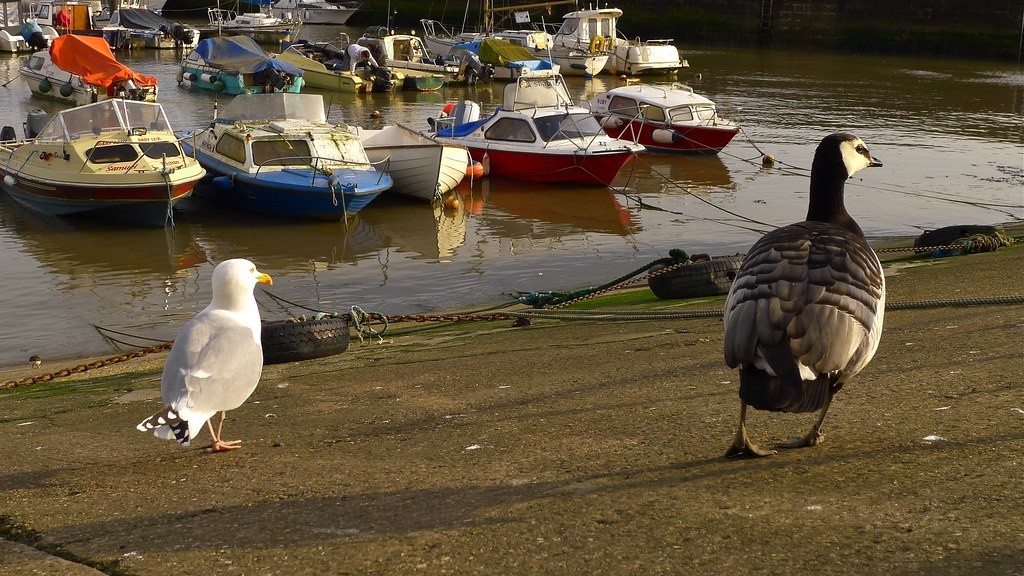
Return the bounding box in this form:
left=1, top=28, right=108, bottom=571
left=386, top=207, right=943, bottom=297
left=134, top=258, right=275, bottom=453
left=719, top=131, right=886, bottom=460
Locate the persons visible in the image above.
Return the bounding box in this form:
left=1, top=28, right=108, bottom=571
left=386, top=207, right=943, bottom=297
left=343, top=44, right=379, bottom=76
left=427, top=117, right=441, bottom=131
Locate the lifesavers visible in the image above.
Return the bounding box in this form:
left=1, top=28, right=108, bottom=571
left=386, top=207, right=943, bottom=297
left=260, top=315, right=353, bottom=365
left=590, top=35, right=605, bottom=53
left=648, top=252, right=744, bottom=299
left=604, top=34, right=614, bottom=51
left=913, top=224, right=999, bottom=256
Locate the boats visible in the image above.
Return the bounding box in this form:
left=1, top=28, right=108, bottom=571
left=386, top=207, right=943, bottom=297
left=17, top=48, right=159, bottom=112
left=341, top=116, right=474, bottom=205
left=0, top=0, right=691, bottom=98
left=424, top=11, right=651, bottom=189
left=172, top=92, right=395, bottom=224
left=560, top=79, right=742, bottom=157
left=0, top=90, right=205, bottom=230
